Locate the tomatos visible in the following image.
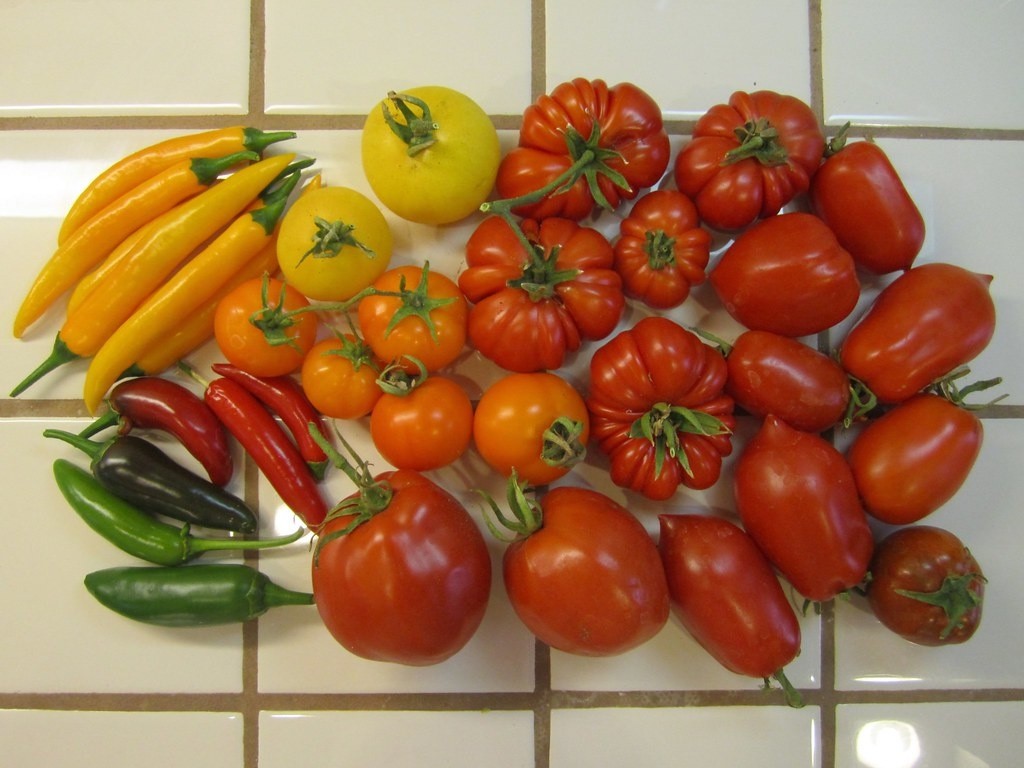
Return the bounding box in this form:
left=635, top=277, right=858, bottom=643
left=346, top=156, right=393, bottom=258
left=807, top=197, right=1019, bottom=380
left=214, top=76, right=1002, bottom=713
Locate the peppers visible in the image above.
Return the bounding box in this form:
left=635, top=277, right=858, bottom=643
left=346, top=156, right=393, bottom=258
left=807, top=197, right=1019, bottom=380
left=10, top=125, right=331, bottom=630
